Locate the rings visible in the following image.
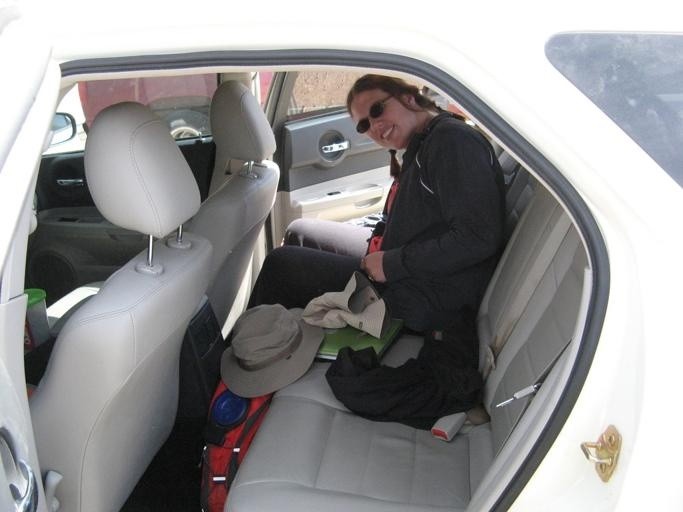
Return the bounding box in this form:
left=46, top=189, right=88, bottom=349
left=368, top=275, right=374, bottom=280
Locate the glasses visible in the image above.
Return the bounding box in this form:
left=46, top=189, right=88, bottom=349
left=356, top=94, right=395, bottom=134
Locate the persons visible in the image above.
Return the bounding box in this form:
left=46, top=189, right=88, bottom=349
left=246, top=73, right=506, bottom=334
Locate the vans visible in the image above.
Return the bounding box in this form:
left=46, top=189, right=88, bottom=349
left=76, top=69, right=274, bottom=142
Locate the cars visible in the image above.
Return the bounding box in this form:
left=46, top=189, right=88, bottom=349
left=0, top=0, right=683, bottom=512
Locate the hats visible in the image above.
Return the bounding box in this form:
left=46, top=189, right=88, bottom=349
left=219, top=302, right=326, bottom=400
left=301, top=270, right=388, bottom=340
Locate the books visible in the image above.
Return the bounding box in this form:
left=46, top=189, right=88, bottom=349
left=316, top=318, right=403, bottom=362
